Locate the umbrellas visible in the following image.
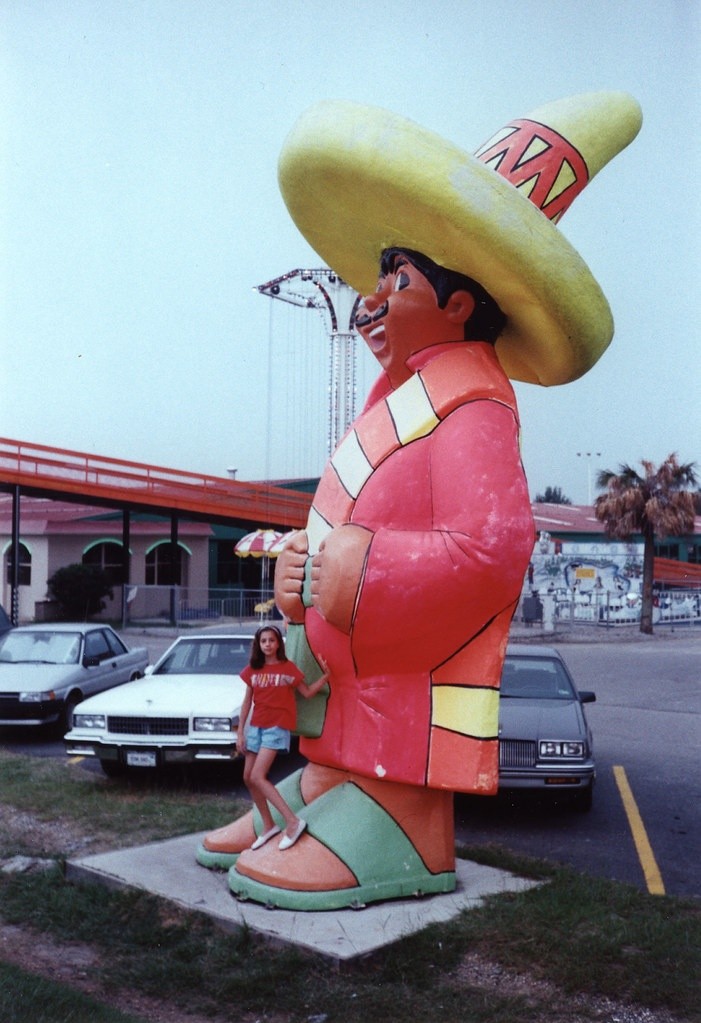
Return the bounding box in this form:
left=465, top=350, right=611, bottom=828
left=268, top=529, right=301, bottom=557
left=233, top=528, right=283, bottom=622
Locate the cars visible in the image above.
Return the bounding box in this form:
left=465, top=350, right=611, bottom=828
left=0, top=621, right=149, bottom=736
left=498, top=643, right=597, bottom=814
left=63, top=635, right=286, bottom=779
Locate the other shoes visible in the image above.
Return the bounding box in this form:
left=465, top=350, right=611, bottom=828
left=279, top=818, right=308, bottom=850
left=251, top=825, right=281, bottom=851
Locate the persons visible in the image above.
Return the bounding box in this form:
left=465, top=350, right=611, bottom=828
left=197, top=90, right=642, bottom=912
left=234, top=625, right=330, bottom=851
